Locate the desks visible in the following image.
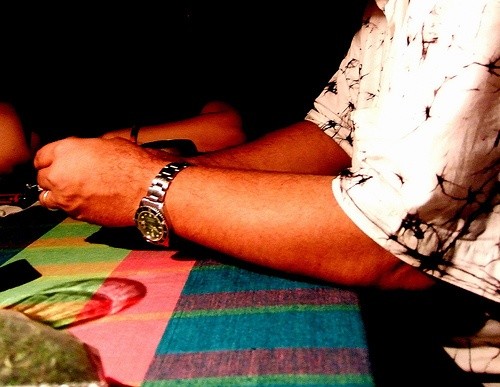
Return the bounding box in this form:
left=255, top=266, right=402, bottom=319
left=0, top=143, right=373, bottom=386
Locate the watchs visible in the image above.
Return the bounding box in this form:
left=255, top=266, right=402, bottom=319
left=133, top=160, right=191, bottom=250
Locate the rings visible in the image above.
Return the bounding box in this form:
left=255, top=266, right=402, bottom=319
left=43, top=190, right=59, bottom=214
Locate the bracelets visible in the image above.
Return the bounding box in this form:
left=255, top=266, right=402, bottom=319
left=129, top=126, right=140, bottom=145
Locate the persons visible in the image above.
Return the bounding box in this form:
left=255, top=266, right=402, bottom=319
left=0, top=0, right=245, bottom=179
left=33, top=0, right=500, bottom=387
